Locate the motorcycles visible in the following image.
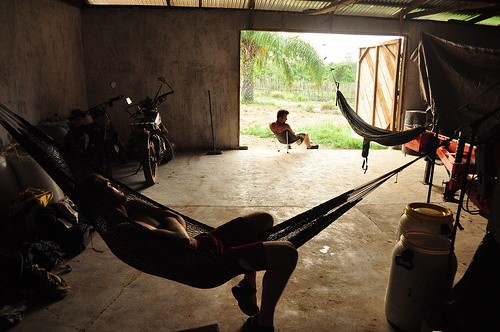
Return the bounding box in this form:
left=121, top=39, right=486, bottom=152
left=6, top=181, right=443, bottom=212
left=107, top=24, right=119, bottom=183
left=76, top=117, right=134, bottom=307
left=124, top=76, right=173, bottom=186
left=77, top=94, right=126, bottom=177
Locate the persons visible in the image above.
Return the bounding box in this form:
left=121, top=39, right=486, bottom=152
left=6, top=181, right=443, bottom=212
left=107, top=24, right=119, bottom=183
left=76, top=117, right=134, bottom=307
left=74, top=173, right=298, bottom=332
left=269, top=109, right=319, bottom=150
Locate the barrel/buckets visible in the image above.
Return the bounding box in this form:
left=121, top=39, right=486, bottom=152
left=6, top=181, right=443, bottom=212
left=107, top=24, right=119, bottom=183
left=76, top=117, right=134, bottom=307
left=396, top=202, right=454, bottom=243
left=385, top=231, right=458, bottom=332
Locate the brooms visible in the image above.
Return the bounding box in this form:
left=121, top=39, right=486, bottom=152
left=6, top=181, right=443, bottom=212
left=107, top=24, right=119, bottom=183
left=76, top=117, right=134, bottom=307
left=206, top=90, right=223, bottom=155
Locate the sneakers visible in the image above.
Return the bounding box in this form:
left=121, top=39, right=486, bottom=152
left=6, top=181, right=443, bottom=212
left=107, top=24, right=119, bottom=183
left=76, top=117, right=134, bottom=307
left=307, top=145, right=318, bottom=149
left=242, top=316, right=274, bottom=332
left=232, top=279, right=259, bottom=316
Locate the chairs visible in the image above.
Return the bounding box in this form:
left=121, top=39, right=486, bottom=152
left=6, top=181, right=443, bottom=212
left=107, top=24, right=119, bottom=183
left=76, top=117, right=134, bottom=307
left=273, top=129, right=300, bottom=154
